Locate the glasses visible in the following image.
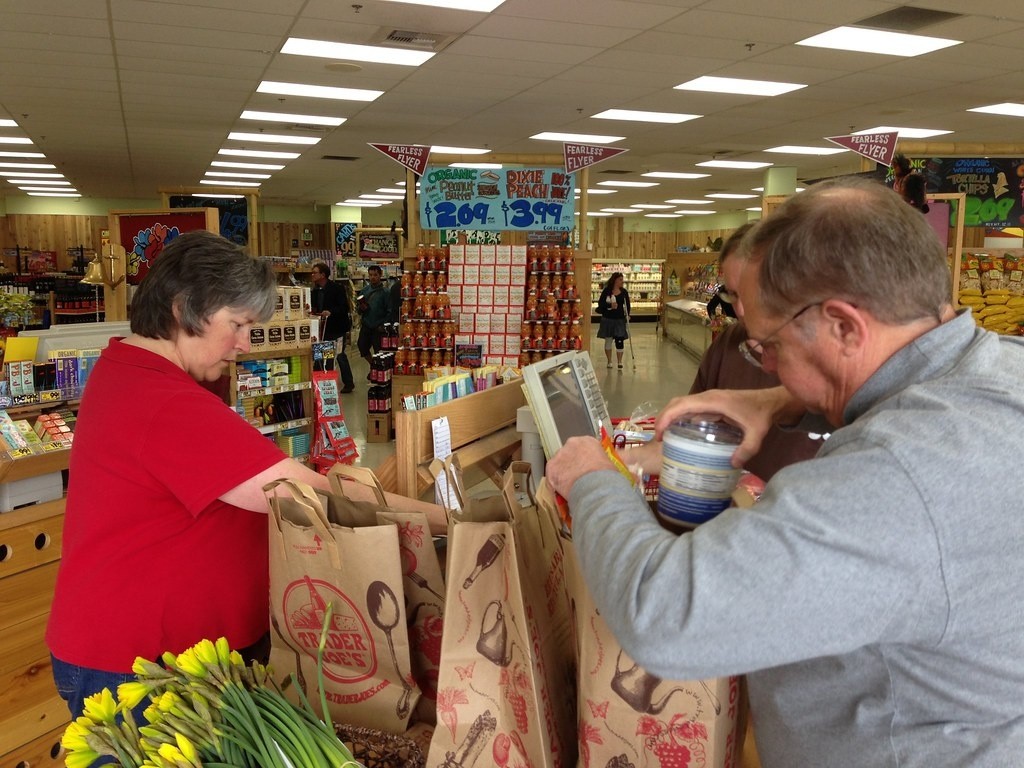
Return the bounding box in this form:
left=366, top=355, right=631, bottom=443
left=311, top=271, right=318, bottom=274
left=738, top=301, right=826, bottom=368
left=715, top=288, right=742, bottom=304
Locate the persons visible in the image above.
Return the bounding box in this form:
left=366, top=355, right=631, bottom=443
left=545, top=176, right=1017, bottom=767
left=309, top=263, right=355, bottom=394
left=890, top=155, right=929, bottom=214
left=46, top=230, right=461, bottom=766
left=355, top=265, right=402, bottom=380
left=595, top=272, right=631, bottom=371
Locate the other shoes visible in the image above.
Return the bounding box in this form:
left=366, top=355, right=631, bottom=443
left=366, top=372, right=371, bottom=379
left=607, top=362, right=613, bottom=368
left=339, top=382, right=356, bottom=395
left=617, top=364, right=625, bottom=371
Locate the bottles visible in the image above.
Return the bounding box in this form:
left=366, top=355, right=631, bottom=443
left=368, top=243, right=582, bottom=413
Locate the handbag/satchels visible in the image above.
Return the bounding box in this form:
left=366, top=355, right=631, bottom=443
left=261, top=461, right=448, bottom=739
left=426, top=452, right=571, bottom=768
left=571, top=536, right=748, bottom=768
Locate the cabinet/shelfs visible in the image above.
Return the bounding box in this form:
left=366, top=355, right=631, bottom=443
left=0, top=186, right=965, bottom=768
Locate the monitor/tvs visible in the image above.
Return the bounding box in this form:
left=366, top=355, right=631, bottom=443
left=539, top=361, right=598, bottom=445
